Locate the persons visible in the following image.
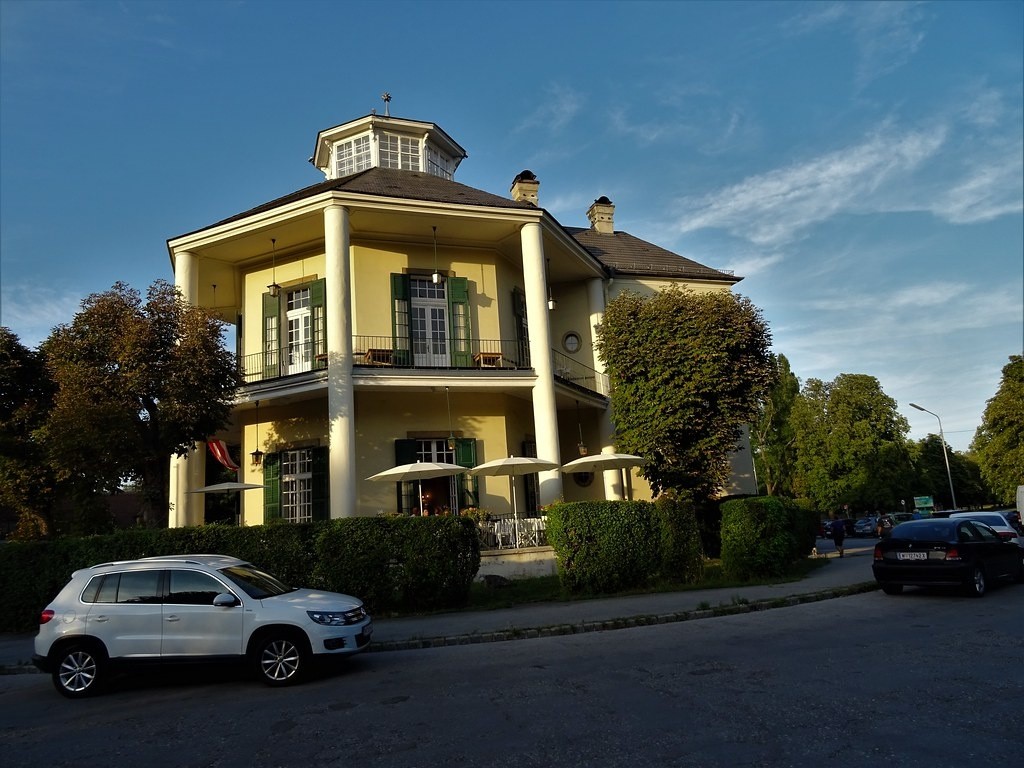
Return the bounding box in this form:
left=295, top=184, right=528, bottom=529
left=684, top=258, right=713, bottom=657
left=410, top=507, right=467, bottom=517
left=877, top=519, right=884, bottom=540
left=863, top=510, right=881, bottom=517
left=831, top=516, right=845, bottom=557
left=910, top=509, right=922, bottom=521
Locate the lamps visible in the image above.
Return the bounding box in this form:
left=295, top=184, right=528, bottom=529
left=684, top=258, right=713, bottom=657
left=249, top=401, right=264, bottom=466
left=575, top=400, right=588, bottom=456
left=431, top=226, right=443, bottom=285
left=547, top=258, right=556, bottom=311
left=445, top=387, right=458, bottom=451
left=267, top=238, right=281, bottom=298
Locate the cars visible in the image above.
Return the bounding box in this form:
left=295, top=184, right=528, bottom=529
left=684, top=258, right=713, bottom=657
left=871, top=517, right=1023, bottom=597
left=819, top=512, right=914, bottom=538
left=949, top=512, right=1021, bottom=547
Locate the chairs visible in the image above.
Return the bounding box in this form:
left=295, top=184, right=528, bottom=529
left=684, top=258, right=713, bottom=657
left=496, top=519, right=515, bottom=550
left=517, top=518, right=539, bottom=548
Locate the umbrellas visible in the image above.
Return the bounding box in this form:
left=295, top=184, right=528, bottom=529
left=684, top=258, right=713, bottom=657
left=469, top=455, right=561, bottom=548
left=364, top=460, right=468, bottom=517
left=562, top=447, right=650, bottom=501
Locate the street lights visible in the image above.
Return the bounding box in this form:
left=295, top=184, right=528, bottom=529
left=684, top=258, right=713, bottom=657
left=908, top=402, right=958, bottom=512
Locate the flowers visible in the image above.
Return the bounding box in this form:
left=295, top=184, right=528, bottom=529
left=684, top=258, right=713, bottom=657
left=537, top=496, right=569, bottom=512
left=462, top=506, right=494, bottom=520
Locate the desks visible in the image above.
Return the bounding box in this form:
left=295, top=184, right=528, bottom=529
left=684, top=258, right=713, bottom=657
left=315, top=353, right=328, bottom=368
left=473, top=352, right=504, bottom=367
left=365, top=348, right=410, bottom=366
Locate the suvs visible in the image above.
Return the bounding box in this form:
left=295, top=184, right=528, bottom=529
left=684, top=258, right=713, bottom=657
left=30, top=554, right=372, bottom=698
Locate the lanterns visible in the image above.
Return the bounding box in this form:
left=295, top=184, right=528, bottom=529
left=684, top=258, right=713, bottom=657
left=578, top=443, right=588, bottom=456
left=448, top=437, right=455, bottom=450
left=250, top=449, right=264, bottom=466
left=432, top=274, right=441, bottom=283
left=547, top=300, right=557, bottom=310
left=268, top=285, right=281, bottom=295
